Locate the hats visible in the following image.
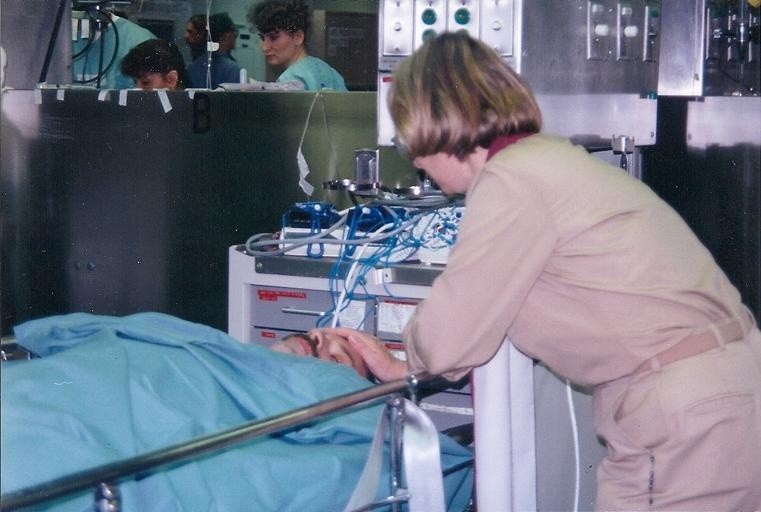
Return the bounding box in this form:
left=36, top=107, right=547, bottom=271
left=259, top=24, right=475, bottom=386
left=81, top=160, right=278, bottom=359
left=203, top=12, right=245, bottom=40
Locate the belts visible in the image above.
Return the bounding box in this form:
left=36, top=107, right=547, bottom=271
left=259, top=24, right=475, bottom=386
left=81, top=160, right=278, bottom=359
left=633, top=312, right=754, bottom=375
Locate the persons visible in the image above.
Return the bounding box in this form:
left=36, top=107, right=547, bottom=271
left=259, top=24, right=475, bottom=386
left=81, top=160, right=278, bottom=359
left=118, top=38, right=189, bottom=91
left=183, top=15, right=238, bottom=64
left=184, top=13, right=241, bottom=90
left=257, top=328, right=377, bottom=386
left=320, top=29, right=761, bottom=512
left=246, top=0, right=349, bottom=92
